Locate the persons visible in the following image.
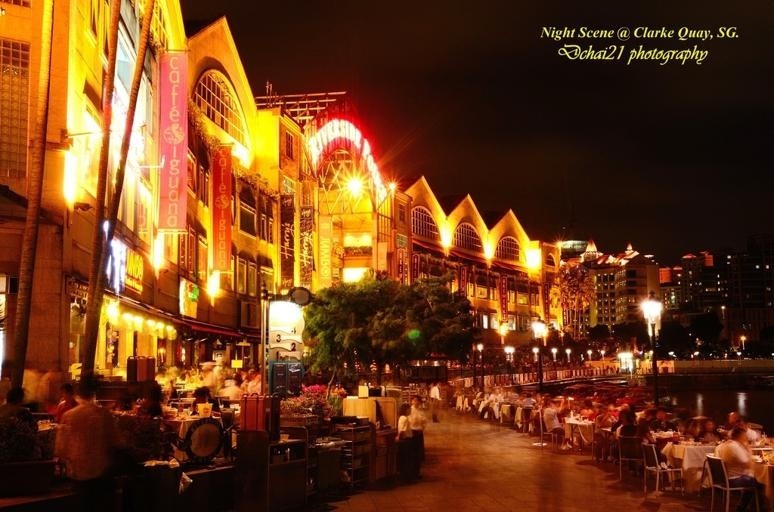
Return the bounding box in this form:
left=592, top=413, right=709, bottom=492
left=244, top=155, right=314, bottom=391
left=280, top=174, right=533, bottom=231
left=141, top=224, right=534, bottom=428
left=0, top=369, right=765, bottom=512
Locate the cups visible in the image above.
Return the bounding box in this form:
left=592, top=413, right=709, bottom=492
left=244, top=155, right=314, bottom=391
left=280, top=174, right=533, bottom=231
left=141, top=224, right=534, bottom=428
left=197, top=403, right=213, bottom=418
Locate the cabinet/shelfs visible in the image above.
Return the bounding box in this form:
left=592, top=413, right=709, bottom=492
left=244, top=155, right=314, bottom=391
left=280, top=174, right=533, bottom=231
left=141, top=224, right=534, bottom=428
left=330, top=424, right=373, bottom=491
left=236, top=426, right=309, bottom=512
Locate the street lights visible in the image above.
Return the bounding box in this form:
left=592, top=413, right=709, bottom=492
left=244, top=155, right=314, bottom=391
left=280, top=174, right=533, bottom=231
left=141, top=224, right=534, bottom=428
left=532, top=316, right=551, bottom=401
left=641, top=290, right=666, bottom=414
left=468, top=334, right=752, bottom=375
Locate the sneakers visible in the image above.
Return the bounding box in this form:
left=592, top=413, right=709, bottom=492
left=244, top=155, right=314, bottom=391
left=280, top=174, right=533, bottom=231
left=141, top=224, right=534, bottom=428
left=561, top=441, right=573, bottom=450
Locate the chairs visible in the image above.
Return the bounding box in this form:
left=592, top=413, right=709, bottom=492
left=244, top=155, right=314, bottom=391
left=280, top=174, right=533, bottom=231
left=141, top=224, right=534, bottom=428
left=539, top=409, right=774, bottom=512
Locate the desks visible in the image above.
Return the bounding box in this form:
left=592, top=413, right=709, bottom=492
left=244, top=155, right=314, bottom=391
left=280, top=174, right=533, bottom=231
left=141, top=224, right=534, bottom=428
left=38, top=423, right=54, bottom=439
left=456, top=394, right=540, bottom=433
left=110, top=406, right=223, bottom=462
